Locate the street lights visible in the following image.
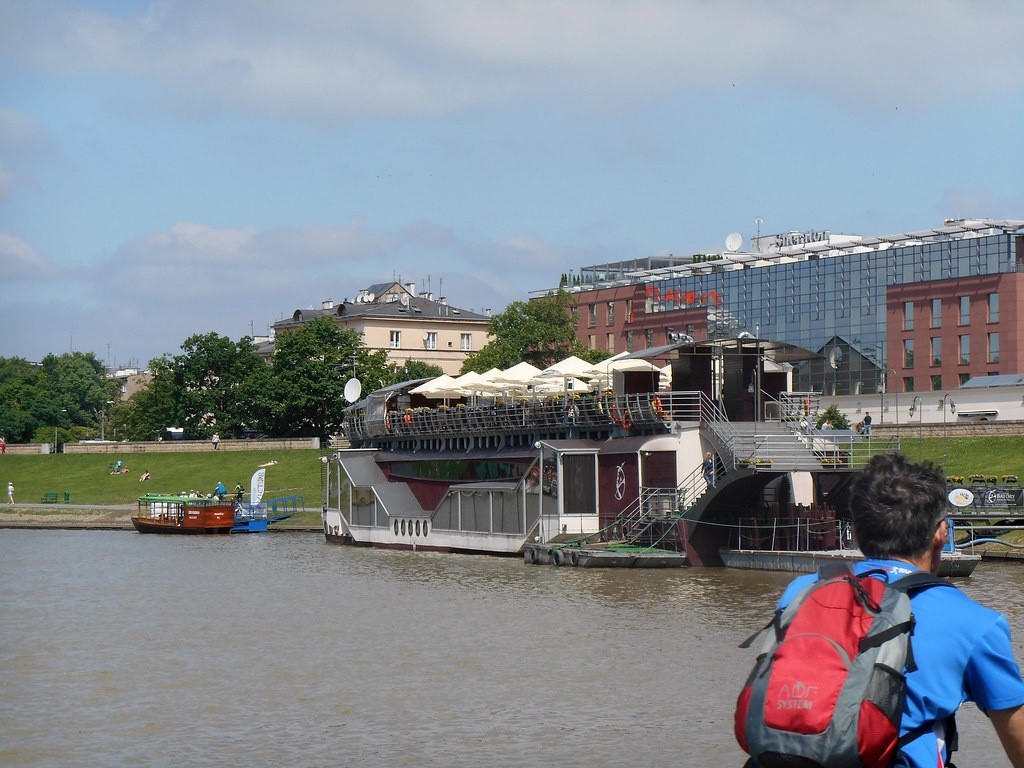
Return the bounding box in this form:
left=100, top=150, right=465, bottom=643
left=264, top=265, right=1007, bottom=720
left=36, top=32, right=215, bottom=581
left=943, top=393, right=956, bottom=483
left=908, top=394, right=922, bottom=465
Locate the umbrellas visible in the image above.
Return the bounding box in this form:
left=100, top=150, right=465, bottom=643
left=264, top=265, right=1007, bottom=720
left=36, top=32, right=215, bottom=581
left=408, top=350, right=672, bottom=407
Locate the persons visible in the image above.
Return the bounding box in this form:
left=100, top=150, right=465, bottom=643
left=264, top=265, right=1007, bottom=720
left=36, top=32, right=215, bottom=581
left=821, top=420, right=833, bottom=430
left=180, top=489, right=219, bottom=501
left=862, top=412, right=872, bottom=438
left=567, top=401, right=579, bottom=426
left=7, top=483, right=15, bottom=504
left=140, top=470, right=150, bottom=481
left=216, top=482, right=226, bottom=494
left=776, top=449, right=1024, bottom=768
left=234, top=483, right=245, bottom=502
left=109, top=460, right=129, bottom=474
left=212, top=431, right=220, bottom=451
left=1, top=442, right=6, bottom=454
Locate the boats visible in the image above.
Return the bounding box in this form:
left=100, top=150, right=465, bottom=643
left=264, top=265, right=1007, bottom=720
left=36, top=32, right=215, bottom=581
left=130, top=493, right=306, bottom=535
left=716, top=516, right=982, bottom=578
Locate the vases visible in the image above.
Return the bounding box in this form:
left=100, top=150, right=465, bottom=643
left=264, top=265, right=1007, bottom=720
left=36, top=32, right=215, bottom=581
left=944, top=479, right=962, bottom=485
left=1004, top=478, right=1017, bottom=484
left=970, top=477, right=996, bottom=483
left=745, top=465, right=772, bottom=468
left=823, top=463, right=840, bottom=468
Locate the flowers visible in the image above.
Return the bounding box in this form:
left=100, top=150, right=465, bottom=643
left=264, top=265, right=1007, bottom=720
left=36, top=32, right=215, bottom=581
left=455, top=404, right=465, bottom=408
left=741, top=456, right=772, bottom=465
left=404, top=408, right=414, bottom=412
left=550, top=396, right=560, bottom=400
left=438, top=406, right=449, bottom=409
left=569, top=395, right=581, bottom=399
left=40, top=494, right=58, bottom=504
left=1001, top=474, right=1018, bottom=483
left=968, top=475, right=997, bottom=482
left=421, top=408, right=431, bottom=411
left=820, top=458, right=842, bottom=465
left=943, top=474, right=963, bottom=484
left=519, top=398, right=529, bottom=402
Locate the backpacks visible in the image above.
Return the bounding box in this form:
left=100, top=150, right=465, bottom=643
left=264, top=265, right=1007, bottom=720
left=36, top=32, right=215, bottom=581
left=734, top=562, right=958, bottom=768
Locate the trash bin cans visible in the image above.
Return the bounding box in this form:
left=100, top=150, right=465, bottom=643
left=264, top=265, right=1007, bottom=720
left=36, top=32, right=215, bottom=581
left=64, top=491, right=69, bottom=504
